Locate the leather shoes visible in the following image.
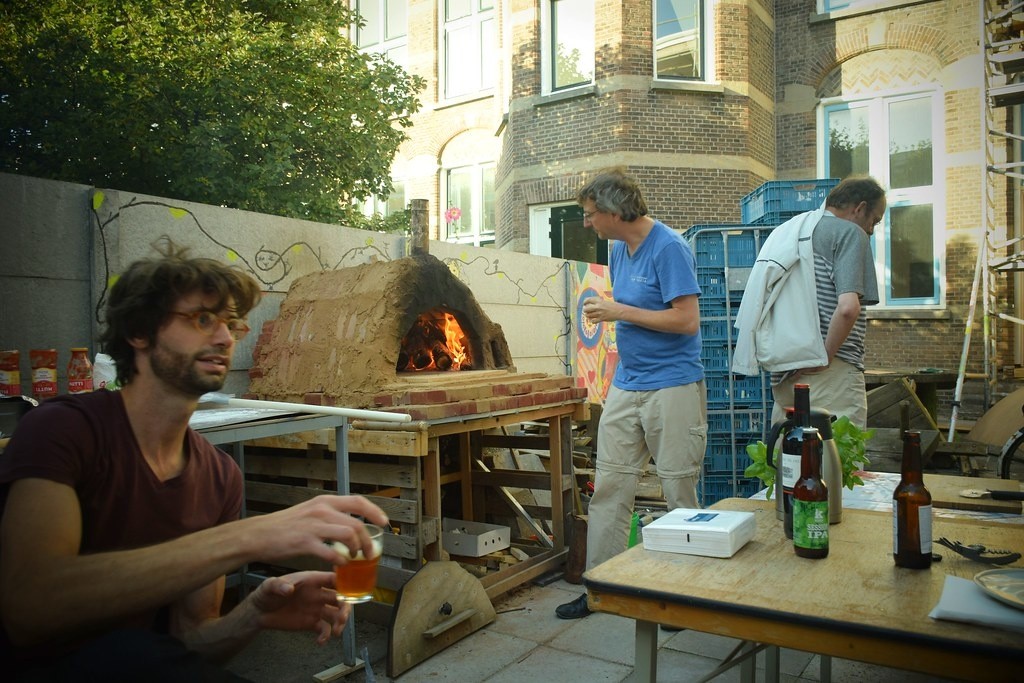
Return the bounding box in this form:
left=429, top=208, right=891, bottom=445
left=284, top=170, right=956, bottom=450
left=555, top=593, right=594, bottom=618
left=660, top=623, right=685, bottom=632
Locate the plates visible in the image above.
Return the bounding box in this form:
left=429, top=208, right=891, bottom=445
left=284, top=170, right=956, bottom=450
left=974, top=568, right=1024, bottom=610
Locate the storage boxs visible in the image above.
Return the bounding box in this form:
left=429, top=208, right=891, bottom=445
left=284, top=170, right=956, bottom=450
left=702, top=373, right=773, bottom=410
left=739, top=177, right=844, bottom=226
left=706, top=410, right=772, bottom=442
left=696, top=474, right=760, bottom=508
left=696, top=307, right=770, bottom=374
left=682, top=224, right=777, bottom=307
left=440, top=517, right=511, bottom=556
left=704, top=440, right=763, bottom=473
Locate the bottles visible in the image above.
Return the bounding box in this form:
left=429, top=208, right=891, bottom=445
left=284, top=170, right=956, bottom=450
left=67, top=347, right=93, bottom=395
left=784, top=384, right=823, bottom=540
left=0, top=350, right=21, bottom=395
left=29, top=349, right=57, bottom=401
left=892, top=431, right=932, bottom=569
left=793, top=428, right=829, bottom=559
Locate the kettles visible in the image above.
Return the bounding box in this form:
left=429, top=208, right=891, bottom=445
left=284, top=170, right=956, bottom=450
left=766, top=406, right=842, bottom=523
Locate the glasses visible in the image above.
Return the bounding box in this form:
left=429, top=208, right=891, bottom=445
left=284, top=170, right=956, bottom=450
left=169, top=311, right=251, bottom=341
left=582, top=207, right=601, bottom=222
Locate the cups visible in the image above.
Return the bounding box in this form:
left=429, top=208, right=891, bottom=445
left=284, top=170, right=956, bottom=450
left=584, top=297, right=599, bottom=322
left=331, top=524, right=384, bottom=604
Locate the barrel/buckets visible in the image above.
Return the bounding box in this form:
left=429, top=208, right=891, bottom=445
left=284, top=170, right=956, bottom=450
left=628, top=513, right=639, bottom=550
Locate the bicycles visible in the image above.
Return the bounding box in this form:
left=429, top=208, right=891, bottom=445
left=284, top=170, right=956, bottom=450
left=997, top=404, right=1024, bottom=480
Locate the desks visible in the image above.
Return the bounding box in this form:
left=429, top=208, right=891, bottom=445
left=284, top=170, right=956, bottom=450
left=188, top=392, right=369, bottom=683
left=582, top=498, right=1024, bottom=683
left=747, top=470, right=1024, bottom=683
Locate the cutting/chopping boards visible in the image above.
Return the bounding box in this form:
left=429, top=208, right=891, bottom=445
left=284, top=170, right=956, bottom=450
left=921, top=474, right=1022, bottom=514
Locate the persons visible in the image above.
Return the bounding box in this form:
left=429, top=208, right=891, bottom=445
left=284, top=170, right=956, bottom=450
left=555, top=172, right=708, bottom=633
left=0, top=257, right=389, bottom=683
left=769, top=177, right=886, bottom=473
